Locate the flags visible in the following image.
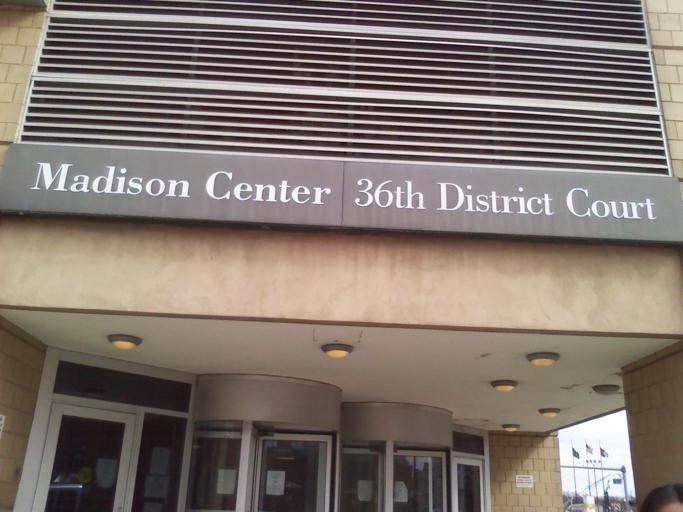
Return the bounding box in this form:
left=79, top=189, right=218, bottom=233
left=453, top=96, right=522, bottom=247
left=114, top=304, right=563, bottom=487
left=585, top=444, right=593, bottom=453
left=572, top=447, right=580, bottom=460
left=599, top=447, right=608, bottom=457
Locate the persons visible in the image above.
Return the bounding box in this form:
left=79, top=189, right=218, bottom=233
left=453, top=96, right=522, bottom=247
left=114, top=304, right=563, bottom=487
left=640, top=481, right=683, bottom=511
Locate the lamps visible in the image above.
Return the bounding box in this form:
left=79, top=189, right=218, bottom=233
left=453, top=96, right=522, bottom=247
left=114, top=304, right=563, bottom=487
left=592, top=385, right=620, bottom=395
left=539, top=408, right=561, bottom=417
left=490, top=380, right=517, bottom=392
left=526, top=351, right=559, bottom=367
left=107, top=334, right=142, bottom=351
left=502, top=424, right=520, bottom=432
left=320, top=343, right=352, bottom=360
